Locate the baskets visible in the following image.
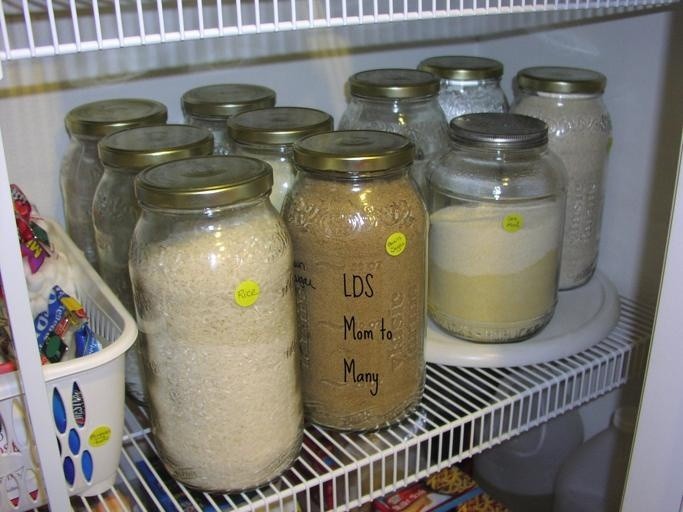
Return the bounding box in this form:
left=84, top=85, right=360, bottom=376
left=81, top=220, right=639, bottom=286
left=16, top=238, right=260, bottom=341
left=0, top=215, right=142, bottom=512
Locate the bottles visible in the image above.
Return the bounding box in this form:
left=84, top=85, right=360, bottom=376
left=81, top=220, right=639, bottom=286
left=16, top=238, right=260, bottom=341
left=59, top=54, right=612, bottom=492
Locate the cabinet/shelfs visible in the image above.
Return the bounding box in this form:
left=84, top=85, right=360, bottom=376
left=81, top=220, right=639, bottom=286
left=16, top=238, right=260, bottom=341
left=0, top=1, right=683, bottom=512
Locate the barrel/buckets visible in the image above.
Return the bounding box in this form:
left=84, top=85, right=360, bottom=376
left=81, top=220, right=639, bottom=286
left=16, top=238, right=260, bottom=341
left=469, top=376, right=637, bottom=512
